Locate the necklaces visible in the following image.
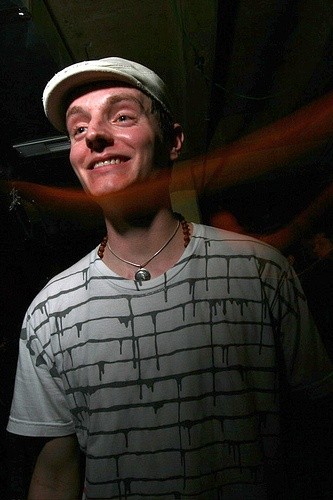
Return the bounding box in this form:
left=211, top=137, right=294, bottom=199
left=97, top=210, right=191, bottom=282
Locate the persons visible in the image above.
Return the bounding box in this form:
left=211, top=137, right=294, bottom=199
left=4, top=55, right=333, bottom=500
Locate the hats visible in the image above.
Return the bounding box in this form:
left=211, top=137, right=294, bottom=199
left=43, top=57, right=176, bottom=136
